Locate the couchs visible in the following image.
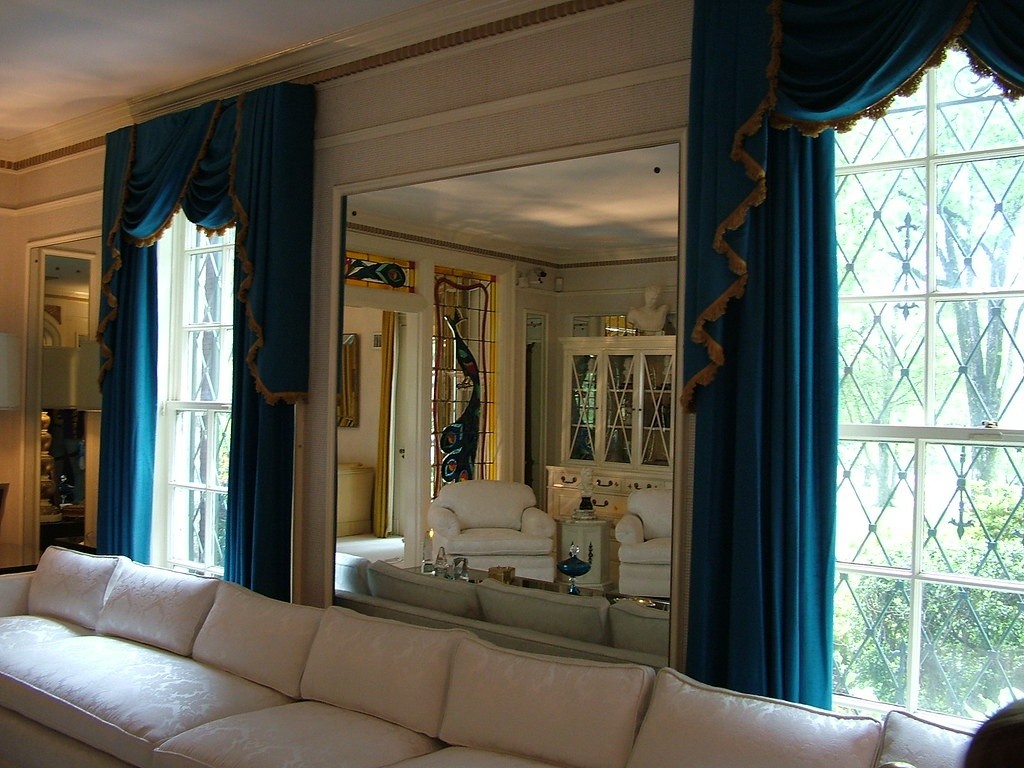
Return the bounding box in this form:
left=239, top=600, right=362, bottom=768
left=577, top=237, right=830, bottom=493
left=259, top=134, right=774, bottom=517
left=0, top=565, right=973, bottom=768
left=336, top=462, right=375, bottom=538
left=334, top=553, right=668, bottom=658
left=428, top=479, right=556, bottom=582
left=615, top=488, right=671, bottom=600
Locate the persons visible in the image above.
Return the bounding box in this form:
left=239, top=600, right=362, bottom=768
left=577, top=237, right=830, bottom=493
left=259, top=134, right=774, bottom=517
left=626, top=285, right=668, bottom=331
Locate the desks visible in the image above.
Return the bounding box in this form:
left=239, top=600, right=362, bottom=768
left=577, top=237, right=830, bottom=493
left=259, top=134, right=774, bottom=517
left=551, top=518, right=614, bottom=596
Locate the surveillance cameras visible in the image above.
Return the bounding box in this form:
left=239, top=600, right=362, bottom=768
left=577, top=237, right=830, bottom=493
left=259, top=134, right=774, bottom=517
left=535, top=267, right=547, bottom=277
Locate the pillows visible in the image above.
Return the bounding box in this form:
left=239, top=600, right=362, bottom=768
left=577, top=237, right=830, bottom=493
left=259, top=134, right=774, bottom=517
left=876, top=709, right=974, bottom=767
left=476, top=577, right=611, bottom=643
left=192, top=580, right=326, bottom=701
left=95, top=560, right=222, bottom=656
left=438, top=635, right=657, bottom=768
left=335, top=552, right=371, bottom=596
left=367, top=560, right=484, bottom=620
left=609, top=602, right=669, bottom=654
left=27, top=546, right=132, bottom=629
left=300, top=606, right=480, bottom=738
left=626, top=667, right=883, bottom=768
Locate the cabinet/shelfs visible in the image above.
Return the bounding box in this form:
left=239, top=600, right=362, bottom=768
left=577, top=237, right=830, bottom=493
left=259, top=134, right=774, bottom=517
left=545, top=334, right=679, bottom=553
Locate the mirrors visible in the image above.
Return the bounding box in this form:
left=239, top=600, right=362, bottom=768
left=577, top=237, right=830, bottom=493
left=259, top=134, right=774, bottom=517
left=335, top=142, right=681, bottom=668
left=39, top=247, right=92, bottom=551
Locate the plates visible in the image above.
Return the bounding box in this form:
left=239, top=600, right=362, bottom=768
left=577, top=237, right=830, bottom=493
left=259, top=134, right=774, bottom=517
left=611, top=597, right=655, bottom=607
